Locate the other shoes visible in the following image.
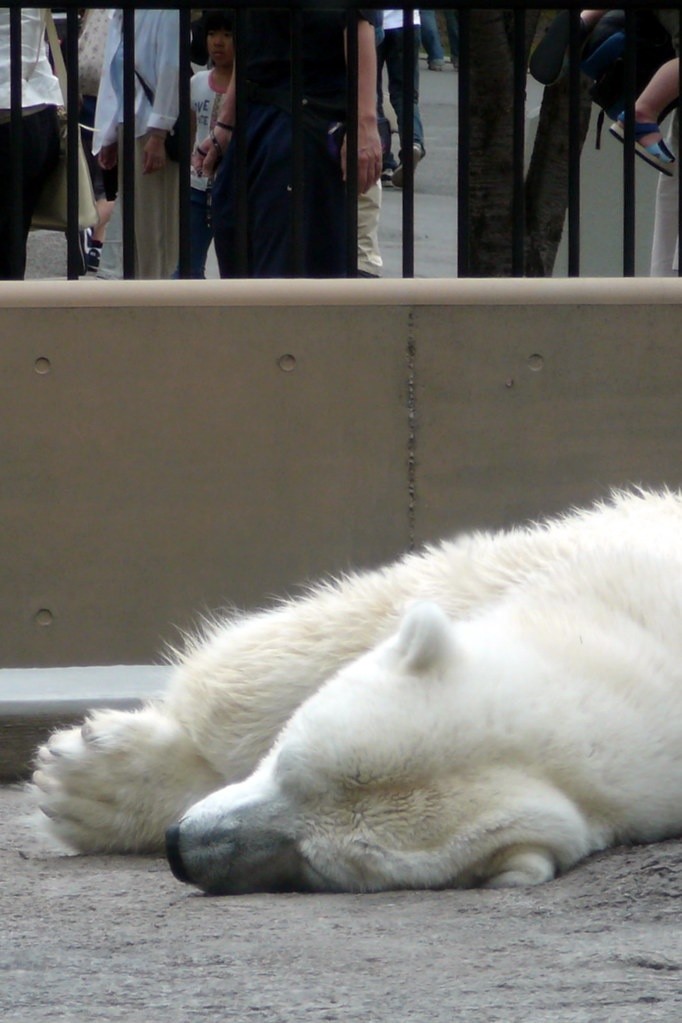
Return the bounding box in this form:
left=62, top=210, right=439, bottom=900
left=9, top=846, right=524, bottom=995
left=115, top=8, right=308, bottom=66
left=66, top=229, right=101, bottom=271
left=381, top=170, right=394, bottom=188
left=451, top=60, right=458, bottom=70
left=392, top=144, right=423, bottom=187
left=428, top=62, right=446, bottom=73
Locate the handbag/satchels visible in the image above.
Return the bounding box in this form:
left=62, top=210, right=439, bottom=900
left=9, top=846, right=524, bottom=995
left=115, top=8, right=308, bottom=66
left=167, top=108, right=197, bottom=161
left=29, top=107, right=101, bottom=232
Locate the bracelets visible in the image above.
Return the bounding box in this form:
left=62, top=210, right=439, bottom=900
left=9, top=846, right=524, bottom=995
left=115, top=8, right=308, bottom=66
left=151, top=132, right=166, bottom=143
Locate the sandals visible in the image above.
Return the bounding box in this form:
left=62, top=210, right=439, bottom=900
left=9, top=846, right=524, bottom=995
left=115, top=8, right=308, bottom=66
left=529, top=11, right=587, bottom=88
left=610, top=113, right=675, bottom=178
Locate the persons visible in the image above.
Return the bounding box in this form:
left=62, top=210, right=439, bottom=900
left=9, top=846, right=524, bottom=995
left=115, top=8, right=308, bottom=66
left=0, top=6, right=681, bottom=277
